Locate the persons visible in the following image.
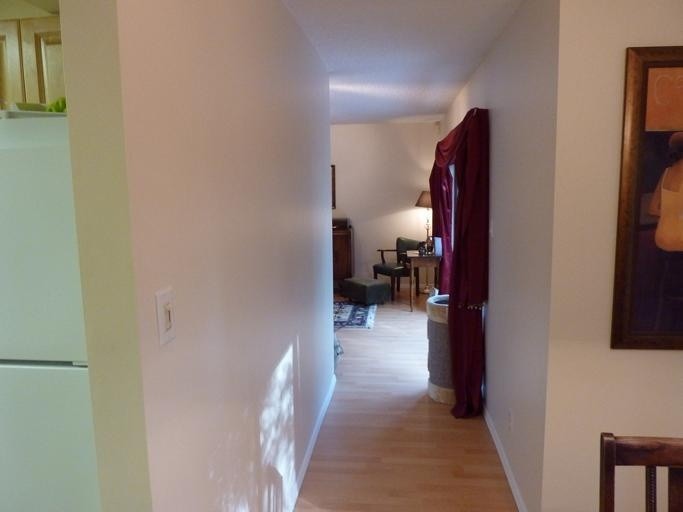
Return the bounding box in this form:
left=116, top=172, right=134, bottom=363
left=647, top=131, right=683, bottom=251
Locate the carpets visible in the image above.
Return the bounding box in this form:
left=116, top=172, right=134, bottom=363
left=333, top=301, right=377, bottom=327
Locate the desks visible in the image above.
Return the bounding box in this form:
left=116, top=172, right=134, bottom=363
left=400, top=251, right=443, bottom=312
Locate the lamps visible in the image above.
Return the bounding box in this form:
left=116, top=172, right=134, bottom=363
left=414, top=191, right=433, bottom=294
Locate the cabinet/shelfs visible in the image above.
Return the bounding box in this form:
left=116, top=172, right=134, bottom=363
left=332, top=218, right=356, bottom=288
left=0, top=15, right=65, bottom=111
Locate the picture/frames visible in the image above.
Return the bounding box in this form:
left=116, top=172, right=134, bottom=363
left=609, top=44, right=683, bottom=351
left=330, top=164, right=336, bottom=209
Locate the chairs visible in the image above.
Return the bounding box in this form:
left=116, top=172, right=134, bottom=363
left=372, top=237, right=425, bottom=301
left=600, top=432, right=683, bottom=512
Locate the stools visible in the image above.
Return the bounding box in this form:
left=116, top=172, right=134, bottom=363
left=344, top=276, right=390, bottom=306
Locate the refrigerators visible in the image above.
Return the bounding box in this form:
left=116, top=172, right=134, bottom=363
left=0, top=116, right=101, bottom=511
